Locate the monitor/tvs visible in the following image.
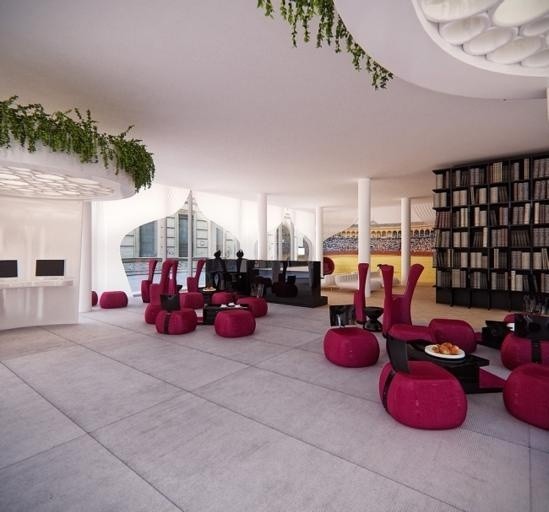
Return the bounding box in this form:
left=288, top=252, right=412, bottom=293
left=35, top=259, right=64, bottom=276
left=0, top=260, right=18, bottom=277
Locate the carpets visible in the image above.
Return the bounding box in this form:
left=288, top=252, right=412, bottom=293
left=0, top=296, right=548, bottom=512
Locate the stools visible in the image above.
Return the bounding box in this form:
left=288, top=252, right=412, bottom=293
left=91, top=291, right=268, bottom=339
left=324, top=307, right=549, bottom=437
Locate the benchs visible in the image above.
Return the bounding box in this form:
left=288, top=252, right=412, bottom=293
left=321, top=272, right=399, bottom=292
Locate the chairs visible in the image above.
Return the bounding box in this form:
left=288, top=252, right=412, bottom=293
left=141, top=258, right=206, bottom=304
left=355, top=264, right=434, bottom=339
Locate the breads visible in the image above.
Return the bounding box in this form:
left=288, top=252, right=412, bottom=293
left=431, top=341, right=461, bottom=355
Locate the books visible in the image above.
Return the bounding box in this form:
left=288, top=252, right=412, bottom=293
left=430, top=154, right=549, bottom=294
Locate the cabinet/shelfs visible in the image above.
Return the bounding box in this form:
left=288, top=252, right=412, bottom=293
left=431, top=152, right=549, bottom=312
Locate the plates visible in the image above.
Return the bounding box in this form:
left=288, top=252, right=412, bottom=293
left=203, top=289, right=217, bottom=292
left=424, top=344, right=465, bottom=359
